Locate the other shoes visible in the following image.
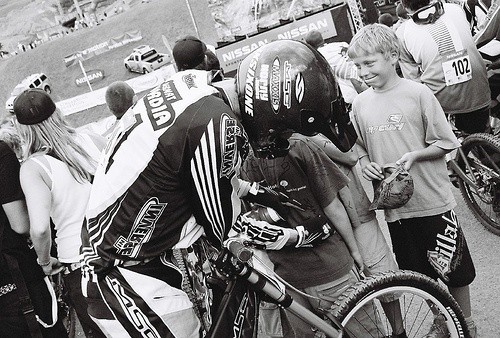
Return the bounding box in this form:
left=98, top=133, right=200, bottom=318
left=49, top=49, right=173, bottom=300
left=465, top=321, right=478, bottom=338
left=426, top=315, right=452, bottom=338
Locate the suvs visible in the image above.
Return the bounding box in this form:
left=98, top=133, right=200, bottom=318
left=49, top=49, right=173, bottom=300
left=6, top=72, right=53, bottom=115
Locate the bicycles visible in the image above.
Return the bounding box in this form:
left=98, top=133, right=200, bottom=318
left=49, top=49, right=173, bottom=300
left=201, top=234, right=472, bottom=338
left=28, top=272, right=77, bottom=338
left=446, top=114, right=500, bottom=239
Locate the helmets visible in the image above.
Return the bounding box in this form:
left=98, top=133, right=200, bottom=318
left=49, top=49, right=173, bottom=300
left=104, top=81, right=138, bottom=119
left=236, top=38, right=358, bottom=162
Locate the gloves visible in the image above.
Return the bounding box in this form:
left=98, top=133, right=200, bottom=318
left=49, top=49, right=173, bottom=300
left=246, top=180, right=307, bottom=222
left=296, top=214, right=336, bottom=249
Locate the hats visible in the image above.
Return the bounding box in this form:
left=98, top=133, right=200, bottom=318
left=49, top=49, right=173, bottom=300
left=173, top=35, right=207, bottom=71
left=367, top=162, right=416, bottom=212
left=12, top=87, right=57, bottom=125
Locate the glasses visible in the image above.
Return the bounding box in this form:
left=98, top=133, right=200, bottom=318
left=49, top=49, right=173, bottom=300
left=406, top=1, right=445, bottom=25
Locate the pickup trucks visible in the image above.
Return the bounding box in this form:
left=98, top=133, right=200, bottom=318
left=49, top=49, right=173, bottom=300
left=123, top=45, right=171, bottom=74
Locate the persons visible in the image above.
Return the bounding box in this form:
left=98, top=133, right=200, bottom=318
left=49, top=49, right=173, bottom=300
left=0, top=0, right=500, bottom=338
left=79, top=36, right=359, bottom=338
left=236, top=129, right=388, bottom=338
left=340, top=20, right=480, bottom=338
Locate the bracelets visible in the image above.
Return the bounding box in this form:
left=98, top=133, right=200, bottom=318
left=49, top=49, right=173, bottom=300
left=358, top=154, right=369, bottom=159
left=36, top=257, right=51, bottom=266
